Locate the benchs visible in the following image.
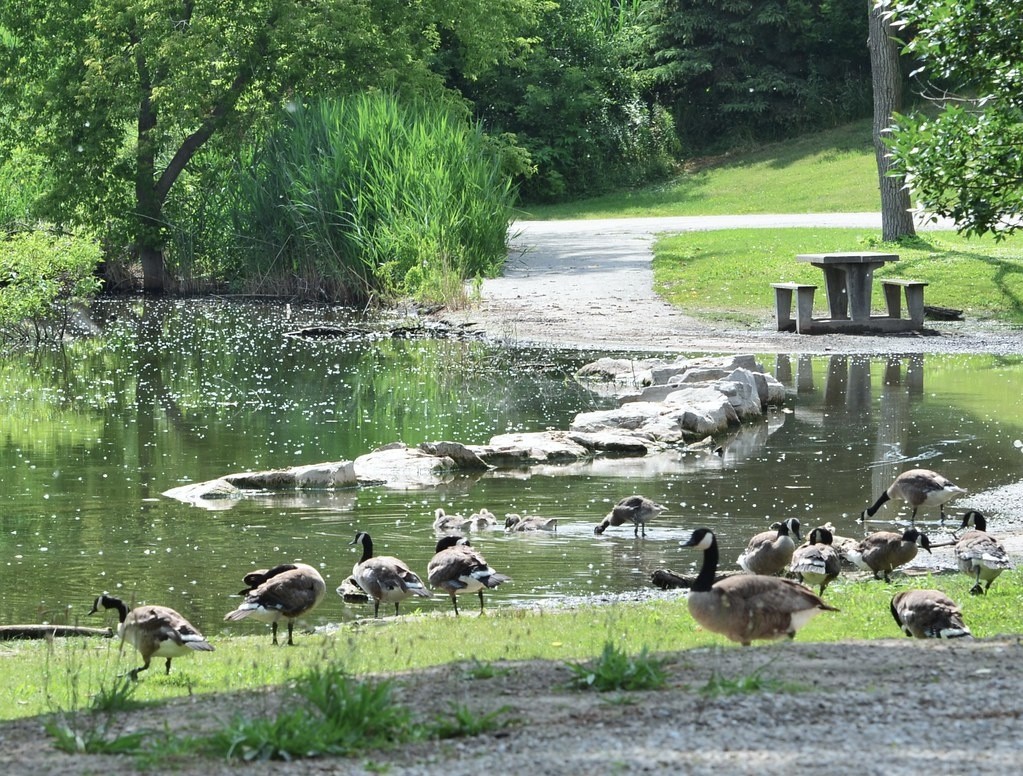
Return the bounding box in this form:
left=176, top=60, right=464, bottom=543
left=879, top=278, right=929, bottom=323
left=769, top=282, right=817, bottom=332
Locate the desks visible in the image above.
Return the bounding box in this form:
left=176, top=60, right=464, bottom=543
left=774, top=252, right=924, bottom=334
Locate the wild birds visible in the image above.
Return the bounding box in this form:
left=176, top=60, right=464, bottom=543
left=891, top=589, right=975, bottom=639
left=594, top=496, right=670, bottom=537
left=680, top=527, right=841, bottom=646
left=427, top=536, right=511, bottom=617
left=87, top=595, right=215, bottom=679
left=955, top=511, right=1015, bottom=595
left=859, top=468, right=966, bottom=527
left=736, top=517, right=932, bottom=613
left=336, top=575, right=370, bottom=602
left=433, top=508, right=557, bottom=531
left=349, top=532, right=432, bottom=619
left=225, top=563, right=327, bottom=645
